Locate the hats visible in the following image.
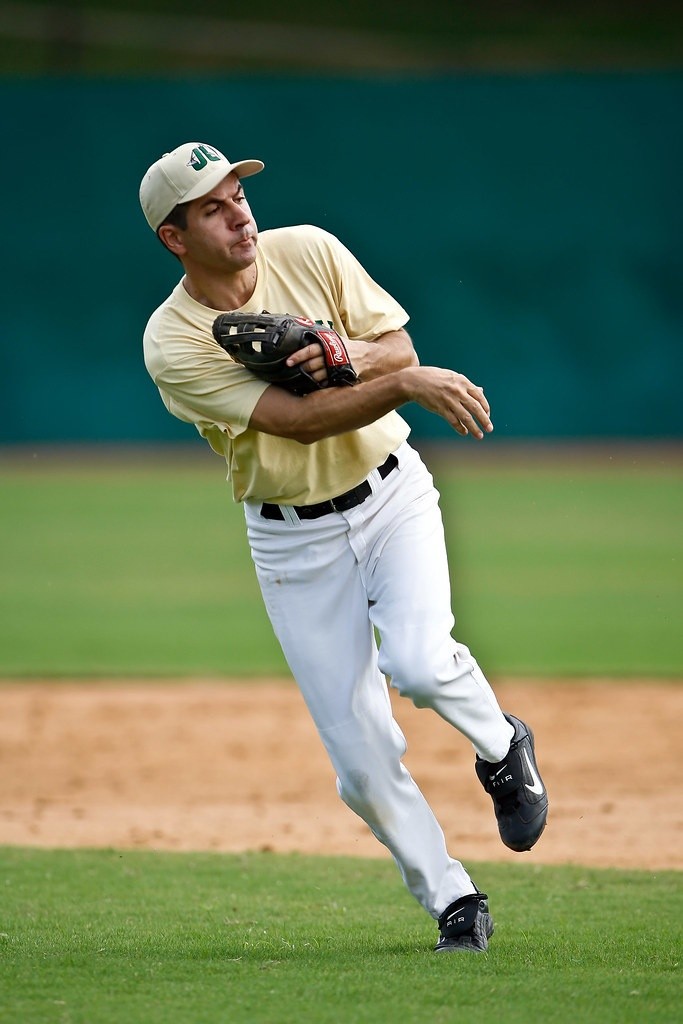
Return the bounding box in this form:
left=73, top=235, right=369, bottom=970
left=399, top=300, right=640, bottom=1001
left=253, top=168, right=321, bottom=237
left=139, top=142, right=265, bottom=233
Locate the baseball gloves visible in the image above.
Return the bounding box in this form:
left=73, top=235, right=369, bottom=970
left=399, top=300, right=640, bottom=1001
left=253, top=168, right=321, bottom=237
left=212, top=310, right=364, bottom=399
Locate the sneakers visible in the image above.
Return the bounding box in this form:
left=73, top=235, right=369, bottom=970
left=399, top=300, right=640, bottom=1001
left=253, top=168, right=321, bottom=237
left=433, top=881, right=495, bottom=954
left=474, top=711, right=549, bottom=852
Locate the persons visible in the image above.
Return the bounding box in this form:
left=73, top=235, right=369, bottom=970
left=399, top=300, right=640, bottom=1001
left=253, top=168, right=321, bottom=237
left=138, top=142, right=548, bottom=953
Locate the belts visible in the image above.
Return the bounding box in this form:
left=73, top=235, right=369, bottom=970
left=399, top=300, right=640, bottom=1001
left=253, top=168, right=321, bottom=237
left=260, top=453, right=398, bottom=520
